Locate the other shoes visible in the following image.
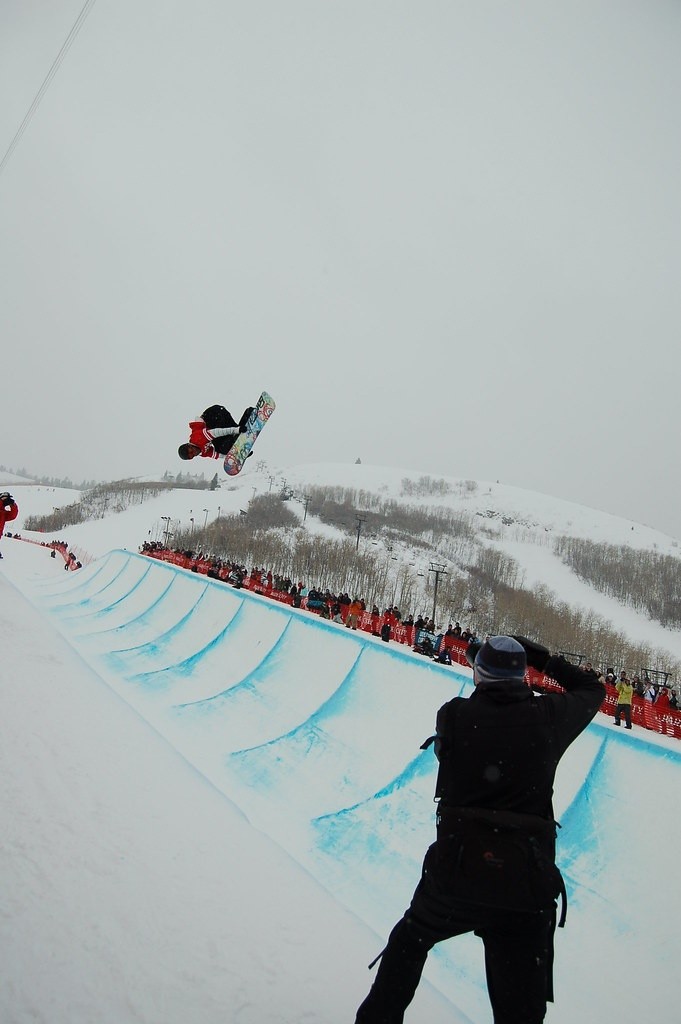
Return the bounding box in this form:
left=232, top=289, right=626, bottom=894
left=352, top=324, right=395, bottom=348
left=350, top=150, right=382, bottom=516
left=352, top=627, right=356, bottom=630
left=624, top=726, right=631, bottom=729
left=346, top=626, right=350, bottom=628
left=613, top=722, right=620, bottom=726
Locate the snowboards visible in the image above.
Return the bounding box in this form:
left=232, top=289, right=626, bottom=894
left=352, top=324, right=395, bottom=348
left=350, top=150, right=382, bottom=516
left=223, top=391, right=276, bottom=476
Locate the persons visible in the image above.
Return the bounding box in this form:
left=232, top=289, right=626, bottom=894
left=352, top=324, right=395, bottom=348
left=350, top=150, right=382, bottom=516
left=5, top=532, right=681, bottom=729
left=178, top=405, right=256, bottom=460
left=0, top=492, right=18, bottom=559
left=352, top=634, right=609, bottom=1024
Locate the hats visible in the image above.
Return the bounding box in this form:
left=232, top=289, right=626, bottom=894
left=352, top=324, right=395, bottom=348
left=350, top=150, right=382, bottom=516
left=625, top=677, right=630, bottom=680
left=644, top=677, right=650, bottom=681
left=607, top=673, right=613, bottom=678
left=474, top=636, right=527, bottom=684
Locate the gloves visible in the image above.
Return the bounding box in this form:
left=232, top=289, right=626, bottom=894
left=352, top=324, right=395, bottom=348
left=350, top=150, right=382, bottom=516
left=3, top=498, right=14, bottom=507
left=511, top=634, right=549, bottom=673
left=239, top=425, right=247, bottom=433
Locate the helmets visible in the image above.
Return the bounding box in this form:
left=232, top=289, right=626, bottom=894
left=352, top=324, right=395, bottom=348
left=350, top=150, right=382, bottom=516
left=178, top=443, right=192, bottom=460
